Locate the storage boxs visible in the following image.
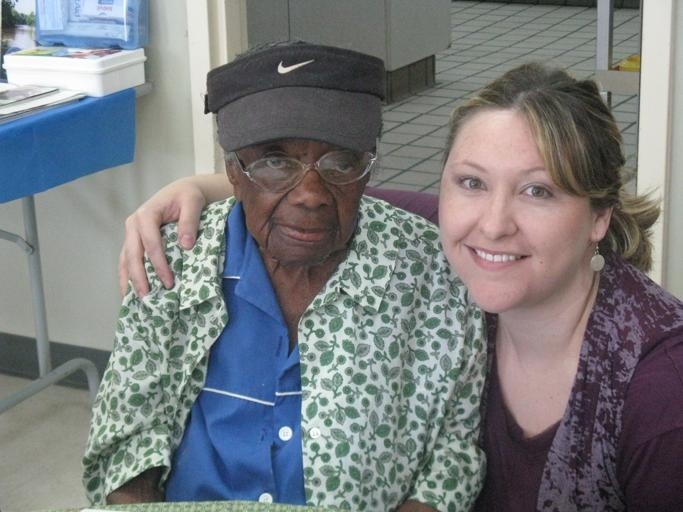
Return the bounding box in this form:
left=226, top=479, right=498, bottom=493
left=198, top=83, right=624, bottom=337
left=2, top=48, right=149, bottom=99
left=34, top=1, right=151, bottom=50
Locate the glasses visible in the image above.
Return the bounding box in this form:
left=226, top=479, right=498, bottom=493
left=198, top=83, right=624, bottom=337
left=233, top=151, right=379, bottom=194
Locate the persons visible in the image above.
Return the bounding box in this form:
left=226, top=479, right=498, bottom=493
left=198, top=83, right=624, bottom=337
left=78, top=35, right=491, bottom=512
left=116, top=58, right=683, bottom=512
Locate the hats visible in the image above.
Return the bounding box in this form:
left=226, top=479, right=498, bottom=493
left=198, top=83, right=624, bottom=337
left=203, top=44, right=389, bottom=154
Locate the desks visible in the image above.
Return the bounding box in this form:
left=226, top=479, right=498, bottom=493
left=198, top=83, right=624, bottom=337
left=0, top=84, right=156, bottom=416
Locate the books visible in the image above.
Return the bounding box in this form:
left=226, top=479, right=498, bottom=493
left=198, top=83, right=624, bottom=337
left=0, top=82, right=86, bottom=126
